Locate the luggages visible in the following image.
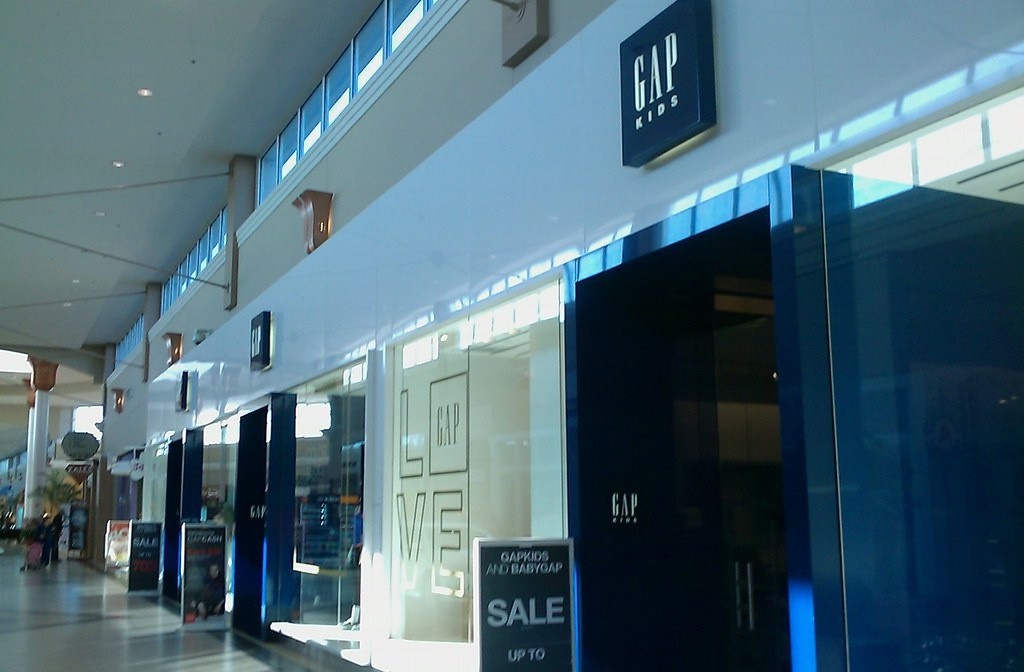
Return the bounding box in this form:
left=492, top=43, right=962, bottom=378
left=25, top=542, right=43, bottom=570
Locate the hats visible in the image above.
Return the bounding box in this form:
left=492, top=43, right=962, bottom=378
left=42, top=512, right=52, bottom=518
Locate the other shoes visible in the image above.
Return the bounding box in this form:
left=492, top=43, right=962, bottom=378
left=337, top=621, right=360, bottom=630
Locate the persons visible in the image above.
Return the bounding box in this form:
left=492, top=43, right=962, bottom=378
left=190, top=563, right=225, bottom=616
left=30, top=507, right=67, bottom=567
